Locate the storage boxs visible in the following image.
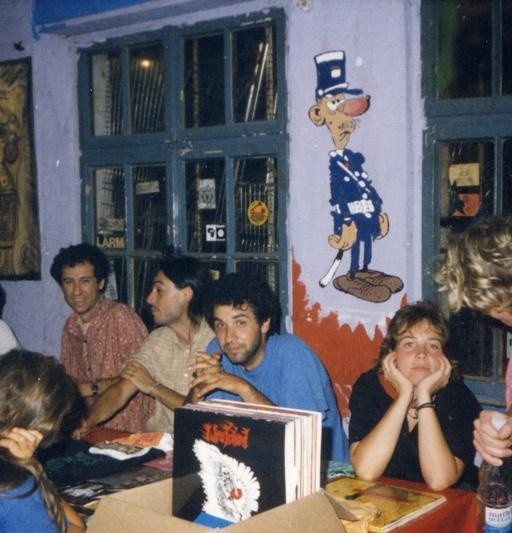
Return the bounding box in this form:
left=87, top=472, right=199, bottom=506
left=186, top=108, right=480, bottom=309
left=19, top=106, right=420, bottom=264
left=87, top=475, right=359, bottom=532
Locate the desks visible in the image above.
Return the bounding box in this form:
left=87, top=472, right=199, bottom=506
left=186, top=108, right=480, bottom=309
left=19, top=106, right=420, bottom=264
left=63, top=427, right=477, bottom=533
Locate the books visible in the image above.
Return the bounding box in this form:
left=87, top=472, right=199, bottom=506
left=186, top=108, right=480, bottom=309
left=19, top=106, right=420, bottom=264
left=171, top=395, right=324, bottom=529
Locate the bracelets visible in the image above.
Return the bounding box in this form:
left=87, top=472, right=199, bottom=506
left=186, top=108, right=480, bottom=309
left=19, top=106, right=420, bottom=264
left=89, top=381, right=101, bottom=393
left=414, top=400, right=436, bottom=411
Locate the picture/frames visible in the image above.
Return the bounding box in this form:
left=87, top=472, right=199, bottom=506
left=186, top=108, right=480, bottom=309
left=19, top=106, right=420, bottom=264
left=0, top=55, right=41, bottom=281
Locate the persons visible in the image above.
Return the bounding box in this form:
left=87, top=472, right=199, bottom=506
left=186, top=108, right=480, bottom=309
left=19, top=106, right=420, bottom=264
left=345, top=298, right=486, bottom=494
left=428, top=214, right=512, bottom=471
left=1, top=346, right=93, bottom=533
left=49, top=241, right=159, bottom=442
left=63, top=252, right=224, bottom=437
left=181, top=268, right=354, bottom=465
left=1, top=284, right=23, bottom=357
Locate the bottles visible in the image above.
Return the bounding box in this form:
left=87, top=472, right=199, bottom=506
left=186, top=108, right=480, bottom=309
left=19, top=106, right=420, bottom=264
left=474, top=414, right=512, bottom=533
